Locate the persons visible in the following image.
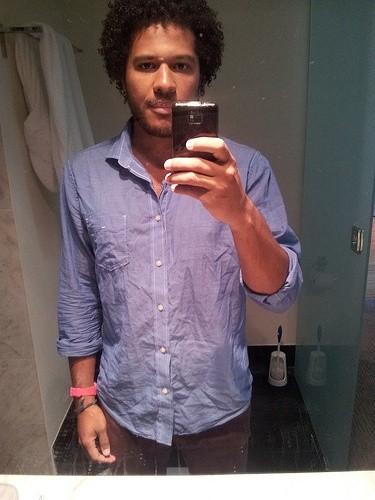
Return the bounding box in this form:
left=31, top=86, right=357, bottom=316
left=56, top=0, right=306, bottom=476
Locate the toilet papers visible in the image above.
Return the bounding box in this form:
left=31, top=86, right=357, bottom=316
left=312, top=272, right=334, bottom=289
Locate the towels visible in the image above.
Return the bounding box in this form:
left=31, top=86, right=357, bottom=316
left=14, top=21, right=96, bottom=194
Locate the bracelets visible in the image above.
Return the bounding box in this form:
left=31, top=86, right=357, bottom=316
left=69, top=382, right=98, bottom=413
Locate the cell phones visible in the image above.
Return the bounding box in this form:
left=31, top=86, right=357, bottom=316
left=172, top=102, right=220, bottom=174
